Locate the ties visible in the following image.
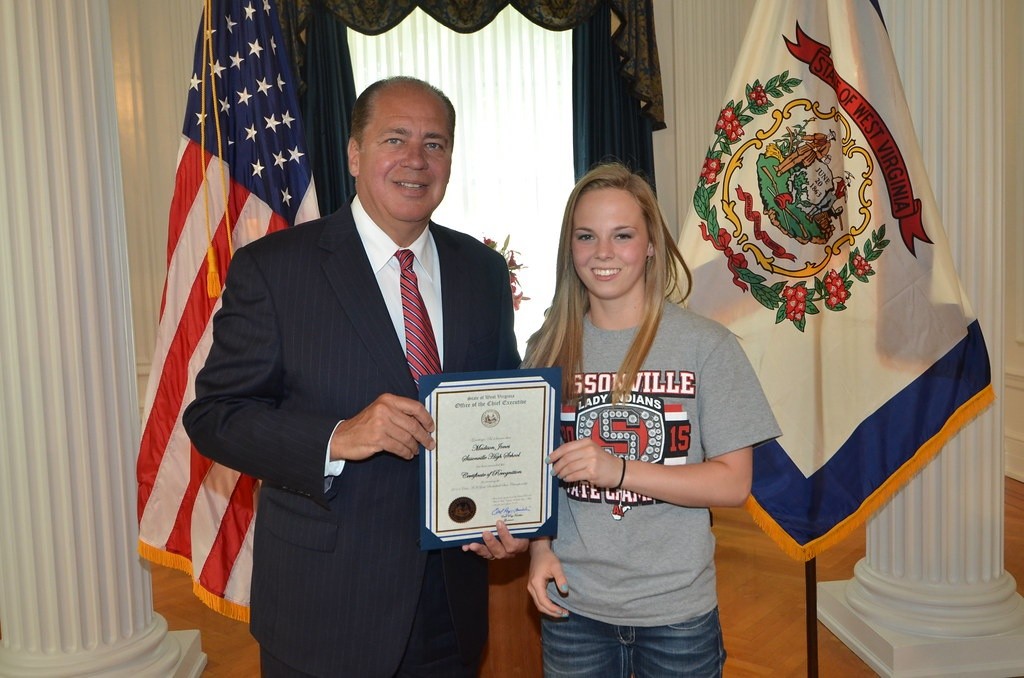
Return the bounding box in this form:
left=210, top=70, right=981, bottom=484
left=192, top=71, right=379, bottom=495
left=392, top=249, right=444, bottom=393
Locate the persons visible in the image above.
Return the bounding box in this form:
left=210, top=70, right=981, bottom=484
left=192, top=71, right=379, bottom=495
left=498, top=160, right=782, bottom=678
left=180, top=76, right=529, bottom=677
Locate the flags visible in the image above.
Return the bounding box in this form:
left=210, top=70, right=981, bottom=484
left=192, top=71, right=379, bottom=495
left=660, top=1, right=998, bottom=561
left=135, top=1, right=330, bottom=631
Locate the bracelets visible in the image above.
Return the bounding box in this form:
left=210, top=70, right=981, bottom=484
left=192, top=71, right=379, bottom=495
left=614, top=457, right=628, bottom=489
